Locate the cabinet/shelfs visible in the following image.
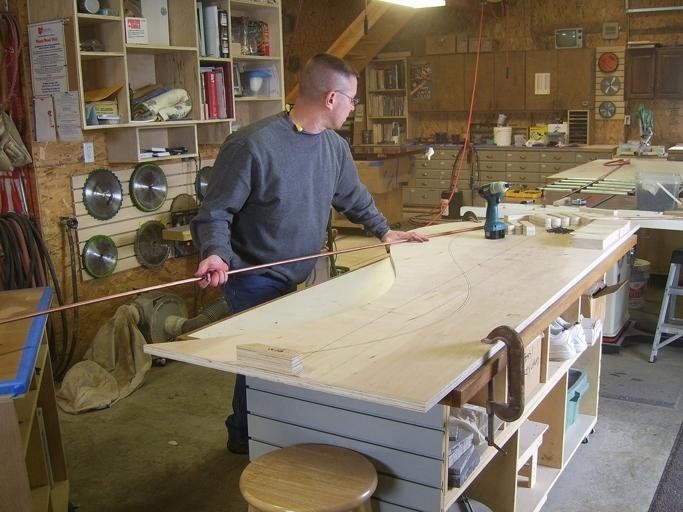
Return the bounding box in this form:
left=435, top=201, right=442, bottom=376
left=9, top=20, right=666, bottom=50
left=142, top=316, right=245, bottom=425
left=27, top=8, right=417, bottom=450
left=625, top=48, right=683, bottom=99
left=141, top=228, right=646, bottom=512
left=522, top=51, right=594, bottom=112
left=25, top=0, right=287, bottom=165
left=474, top=150, right=613, bottom=206
left=0, top=287, right=73, bottom=511
left=404, top=146, right=469, bottom=209
left=407, top=56, right=466, bottom=112
left=465, top=54, right=524, bottom=111
left=346, top=55, right=410, bottom=163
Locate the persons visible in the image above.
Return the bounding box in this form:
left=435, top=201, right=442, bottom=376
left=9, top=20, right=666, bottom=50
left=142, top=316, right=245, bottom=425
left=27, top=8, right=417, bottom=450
left=188, top=51, right=428, bottom=456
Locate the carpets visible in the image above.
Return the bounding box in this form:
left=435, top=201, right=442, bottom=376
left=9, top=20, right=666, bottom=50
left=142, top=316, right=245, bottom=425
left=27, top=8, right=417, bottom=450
left=648, top=422, right=681, bottom=512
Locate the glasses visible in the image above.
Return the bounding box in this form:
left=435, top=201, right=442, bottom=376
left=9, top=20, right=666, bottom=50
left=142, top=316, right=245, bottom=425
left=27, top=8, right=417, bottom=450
left=334, top=88, right=360, bottom=106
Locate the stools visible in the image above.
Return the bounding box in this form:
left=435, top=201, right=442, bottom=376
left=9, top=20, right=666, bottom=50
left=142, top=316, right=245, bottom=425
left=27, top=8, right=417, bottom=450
left=516, top=418, right=549, bottom=488
left=238, top=441, right=380, bottom=512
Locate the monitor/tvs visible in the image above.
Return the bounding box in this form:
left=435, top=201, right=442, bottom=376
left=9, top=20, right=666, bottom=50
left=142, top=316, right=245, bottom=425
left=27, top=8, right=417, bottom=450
left=554, top=28, right=583, bottom=49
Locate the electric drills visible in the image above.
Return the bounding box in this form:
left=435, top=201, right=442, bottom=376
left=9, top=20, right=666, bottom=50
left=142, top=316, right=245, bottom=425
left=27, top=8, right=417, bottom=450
left=478, top=180, right=513, bottom=240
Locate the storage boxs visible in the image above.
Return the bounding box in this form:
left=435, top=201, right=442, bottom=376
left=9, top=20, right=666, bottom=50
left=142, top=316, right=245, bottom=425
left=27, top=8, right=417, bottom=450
left=353, top=156, right=405, bottom=225
left=563, top=366, right=589, bottom=430
left=525, top=334, right=541, bottom=401
left=633, top=171, right=680, bottom=212
left=424, top=33, right=498, bottom=54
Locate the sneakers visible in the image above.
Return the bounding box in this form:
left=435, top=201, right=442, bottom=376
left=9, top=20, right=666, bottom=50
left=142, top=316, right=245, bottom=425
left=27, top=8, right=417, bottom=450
left=227, top=439, right=248, bottom=455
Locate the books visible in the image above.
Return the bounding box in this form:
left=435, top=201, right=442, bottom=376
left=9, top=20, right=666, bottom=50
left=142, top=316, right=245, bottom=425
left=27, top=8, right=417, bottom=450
left=195, top=2, right=269, bottom=123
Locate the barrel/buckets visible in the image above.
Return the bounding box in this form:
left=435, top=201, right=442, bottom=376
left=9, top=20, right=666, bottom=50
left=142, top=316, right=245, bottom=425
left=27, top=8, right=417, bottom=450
left=495, top=126, right=512, bottom=146
left=629, top=258, right=650, bottom=309
left=441, top=191, right=462, bottom=218
